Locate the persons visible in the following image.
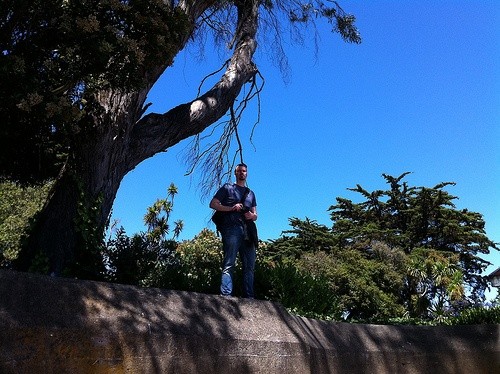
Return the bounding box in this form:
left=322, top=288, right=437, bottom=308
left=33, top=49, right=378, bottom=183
left=209, top=164, right=257, bottom=299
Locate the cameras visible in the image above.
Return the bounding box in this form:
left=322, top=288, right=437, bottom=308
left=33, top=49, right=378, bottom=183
left=240, top=207, right=248, bottom=213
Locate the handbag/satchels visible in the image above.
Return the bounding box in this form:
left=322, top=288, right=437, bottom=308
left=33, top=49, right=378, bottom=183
left=212, top=188, right=250, bottom=232
